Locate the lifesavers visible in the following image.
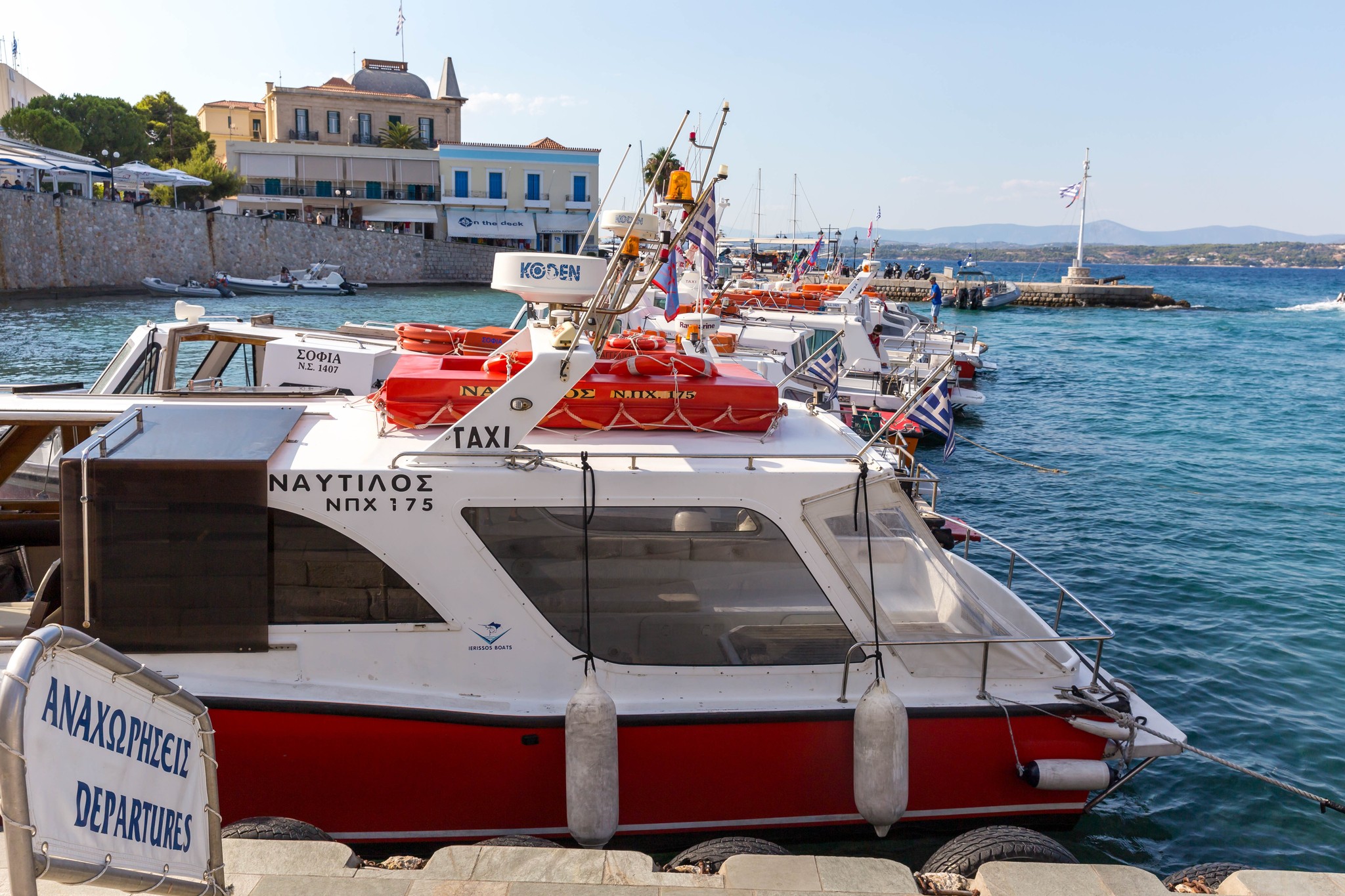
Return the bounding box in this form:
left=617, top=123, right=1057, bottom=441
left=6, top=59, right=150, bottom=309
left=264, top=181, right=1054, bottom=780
left=744, top=290, right=819, bottom=300
left=606, top=329, right=667, bottom=350
left=954, top=286, right=958, bottom=296
left=208, top=278, right=216, bottom=287
left=394, top=322, right=471, bottom=355
left=809, top=291, right=834, bottom=300
left=985, top=287, right=991, bottom=297
left=472, top=833, right=566, bottom=848
left=1161, top=862, right=1257, bottom=890
left=918, top=824, right=1082, bottom=880
left=608, top=353, right=718, bottom=377
left=699, top=298, right=736, bottom=306
left=479, top=350, right=595, bottom=375
left=741, top=272, right=753, bottom=279
left=864, top=286, right=876, bottom=292
left=220, top=815, right=335, bottom=842
left=669, top=836, right=795, bottom=870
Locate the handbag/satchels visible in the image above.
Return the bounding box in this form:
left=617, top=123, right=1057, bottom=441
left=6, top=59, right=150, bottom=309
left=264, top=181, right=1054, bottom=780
left=321, top=221, right=325, bottom=224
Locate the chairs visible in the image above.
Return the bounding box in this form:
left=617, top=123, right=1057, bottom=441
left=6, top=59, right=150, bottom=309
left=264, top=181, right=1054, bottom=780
left=385, top=190, right=395, bottom=199
left=284, top=187, right=293, bottom=195
left=251, top=185, right=260, bottom=194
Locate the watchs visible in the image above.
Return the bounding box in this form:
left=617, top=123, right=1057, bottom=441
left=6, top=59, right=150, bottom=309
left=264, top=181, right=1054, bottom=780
left=926, top=298, right=929, bottom=301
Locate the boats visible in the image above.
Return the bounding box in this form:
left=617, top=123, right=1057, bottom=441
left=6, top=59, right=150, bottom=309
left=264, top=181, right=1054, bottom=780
left=140, top=273, right=236, bottom=299
left=210, top=247, right=359, bottom=296
left=0, top=96, right=1190, bottom=854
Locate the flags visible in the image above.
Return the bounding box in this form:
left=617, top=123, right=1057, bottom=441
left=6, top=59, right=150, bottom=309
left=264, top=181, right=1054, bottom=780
left=651, top=183, right=718, bottom=322
left=836, top=258, right=842, bottom=275
left=793, top=235, right=823, bottom=285
left=1059, top=177, right=1086, bottom=208
left=868, top=220, right=873, bottom=238
left=805, top=341, right=838, bottom=403
left=876, top=205, right=881, bottom=221
left=904, top=373, right=956, bottom=463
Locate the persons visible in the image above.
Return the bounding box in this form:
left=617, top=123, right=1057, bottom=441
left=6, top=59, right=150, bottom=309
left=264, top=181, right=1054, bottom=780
left=868, top=324, right=887, bottom=367
left=271, top=210, right=275, bottom=219
left=248, top=208, right=256, bottom=216
left=923, top=276, right=942, bottom=327
left=316, top=211, right=345, bottom=227
left=773, top=255, right=778, bottom=273
left=1, top=179, right=48, bottom=194
left=103, top=189, right=162, bottom=206
left=507, top=238, right=531, bottom=250
left=856, top=264, right=862, bottom=272
left=472, top=238, right=506, bottom=246
left=360, top=220, right=374, bottom=231
left=386, top=222, right=411, bottom=235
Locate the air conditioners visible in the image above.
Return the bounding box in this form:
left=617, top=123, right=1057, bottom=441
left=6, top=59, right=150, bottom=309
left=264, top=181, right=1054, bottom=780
left=299, top=188, right=308, bottom=195
left=396, top=192, right=405, bottom=199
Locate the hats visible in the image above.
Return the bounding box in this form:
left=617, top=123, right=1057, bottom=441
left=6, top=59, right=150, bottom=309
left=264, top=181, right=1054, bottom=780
left=927, top=276, right=936, bottom=280
left=26, top=182, right=32, bottom=185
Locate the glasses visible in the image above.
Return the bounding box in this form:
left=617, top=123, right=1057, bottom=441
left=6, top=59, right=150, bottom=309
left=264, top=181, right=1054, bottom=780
left=930, top=279, right=935, bottom=281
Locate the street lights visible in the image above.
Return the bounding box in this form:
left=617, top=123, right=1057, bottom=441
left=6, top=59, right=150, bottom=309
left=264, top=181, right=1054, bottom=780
left=101, top=149, right=120, bottom=202
left=335, top=189, right=352, bottom=228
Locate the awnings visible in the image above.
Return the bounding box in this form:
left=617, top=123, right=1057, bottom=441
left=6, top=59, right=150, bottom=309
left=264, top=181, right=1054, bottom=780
left=362, top=203, right=438, bottom=223
left=446, top=209, right=537, bottom=239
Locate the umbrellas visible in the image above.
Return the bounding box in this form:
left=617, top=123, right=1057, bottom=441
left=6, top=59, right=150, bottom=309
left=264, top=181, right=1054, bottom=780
left=106, top=161, right=212, bottom=208
